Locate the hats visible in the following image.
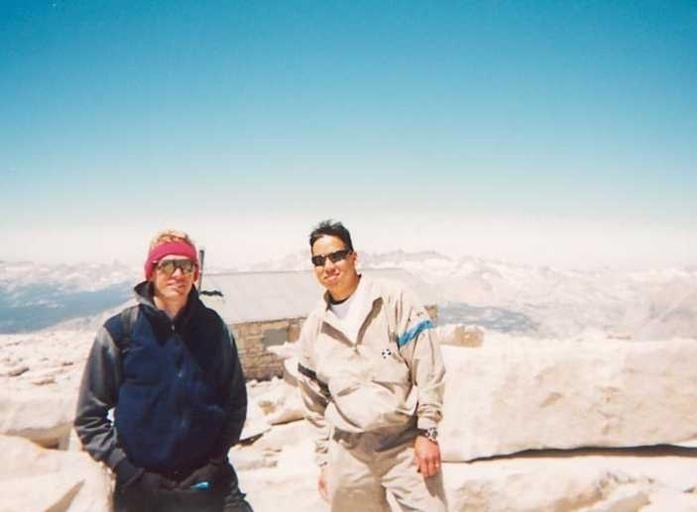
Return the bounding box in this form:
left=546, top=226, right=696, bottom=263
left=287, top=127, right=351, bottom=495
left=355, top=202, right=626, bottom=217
left=145, top=230, right=201, bottom=284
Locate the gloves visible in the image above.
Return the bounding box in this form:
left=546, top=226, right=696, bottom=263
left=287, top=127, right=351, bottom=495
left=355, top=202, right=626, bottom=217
left=114, top=467, right=173, bottom=505
left=172, top=465, right=220, bottom=503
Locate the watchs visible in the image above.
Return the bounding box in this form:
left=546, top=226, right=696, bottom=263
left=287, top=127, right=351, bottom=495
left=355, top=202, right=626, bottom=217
left=416, top=428, right=440, bottom=442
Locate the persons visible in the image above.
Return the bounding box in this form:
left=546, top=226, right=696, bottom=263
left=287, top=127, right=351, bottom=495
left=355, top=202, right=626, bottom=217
left=72, top=230, right=254, bottom=512
left=296, top=221, right=451, bottom=512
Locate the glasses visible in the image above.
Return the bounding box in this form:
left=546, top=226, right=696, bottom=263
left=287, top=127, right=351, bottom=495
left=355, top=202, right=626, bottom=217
left=311, top=248, right=349, bottom=266
left=155, top=257, right=196, bottom=274
